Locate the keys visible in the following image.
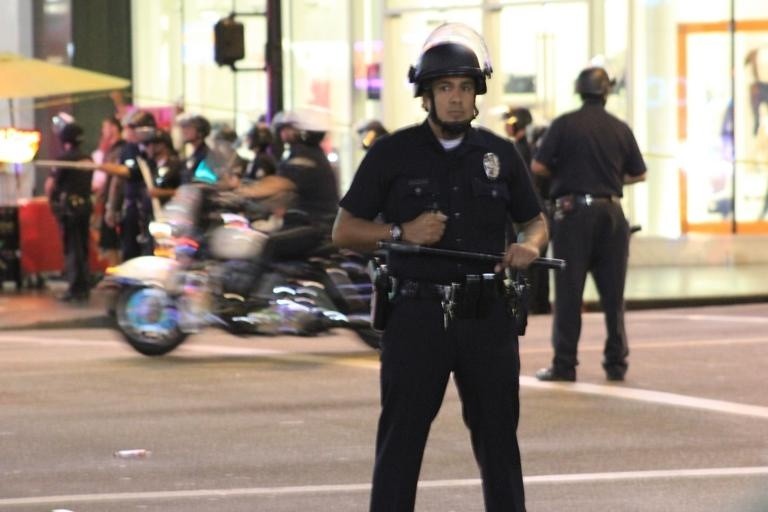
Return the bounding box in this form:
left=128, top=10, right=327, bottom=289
left=440, top=299, right=455, bottom=330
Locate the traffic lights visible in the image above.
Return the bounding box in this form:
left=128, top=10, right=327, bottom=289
left=212, top=21, right=248, bottom=66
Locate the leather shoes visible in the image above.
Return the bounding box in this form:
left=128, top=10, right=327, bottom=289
left=607, top=371, right=625, bottom=381
left=537, top=364, right=576, bottom=381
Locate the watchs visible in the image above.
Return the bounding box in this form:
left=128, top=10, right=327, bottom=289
left=394, top=224, right=403, bottom=241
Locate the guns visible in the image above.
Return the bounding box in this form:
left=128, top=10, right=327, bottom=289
left=365, top=260, right=393, bottom=331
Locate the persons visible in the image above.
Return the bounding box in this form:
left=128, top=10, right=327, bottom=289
left=504, top=107, right=554, bottom=312
left=530, top=70, right=647, bottom=384
left=333, top=42, right=549, bottom=511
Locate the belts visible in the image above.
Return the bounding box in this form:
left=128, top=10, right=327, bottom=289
left=391, top=277, right=508, bottom=304
left=558, top=193, right=620, bottom=207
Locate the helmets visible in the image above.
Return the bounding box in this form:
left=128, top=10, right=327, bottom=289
left=502, top=109, right=534, bottom=134
left=127, top=107, right=330, bottom=145
left=406, top=23, right=493, bottom=98
left=53, top=113, right=84, bottom=144
left=573, top=66, right=618, bottom=98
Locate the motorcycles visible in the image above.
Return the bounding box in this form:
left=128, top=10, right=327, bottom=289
left=98, top=168, right=393, bottom=352
